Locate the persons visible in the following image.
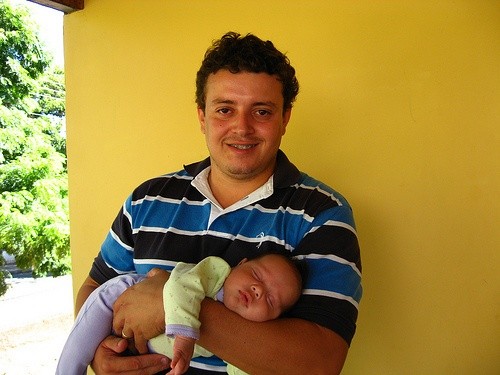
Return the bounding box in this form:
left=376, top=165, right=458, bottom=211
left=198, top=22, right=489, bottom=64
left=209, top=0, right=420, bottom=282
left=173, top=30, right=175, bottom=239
left=56, top=252, right=302, bottom=375
left=74, top=30, right=363, bottom=375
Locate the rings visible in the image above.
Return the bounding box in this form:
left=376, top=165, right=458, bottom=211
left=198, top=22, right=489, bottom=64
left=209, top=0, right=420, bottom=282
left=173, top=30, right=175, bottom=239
left=121, top=330, right=132, bottom=339
